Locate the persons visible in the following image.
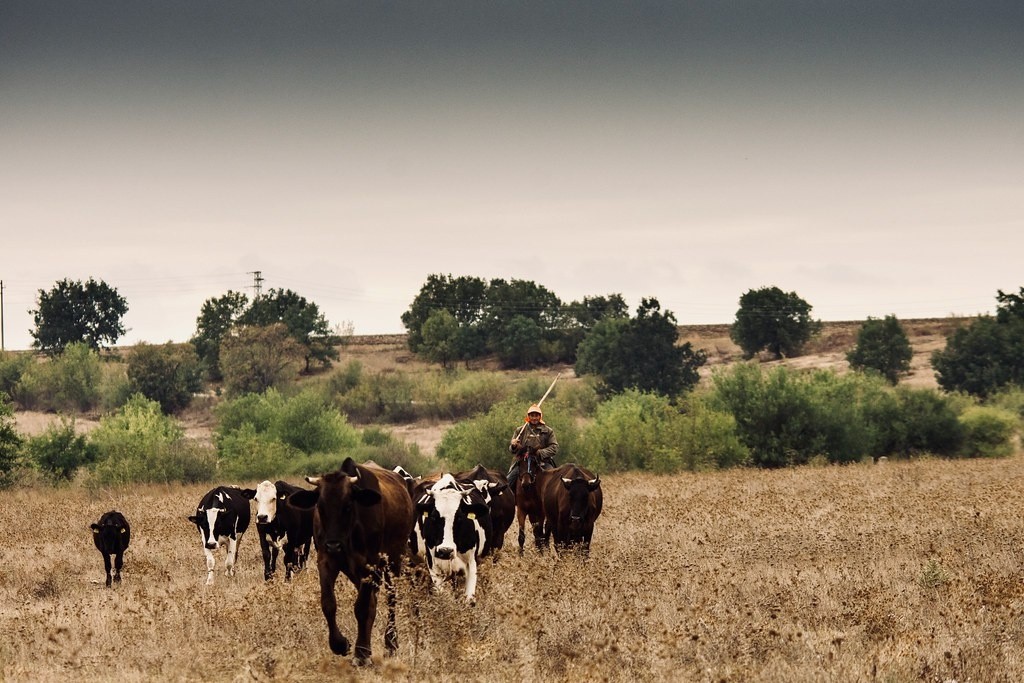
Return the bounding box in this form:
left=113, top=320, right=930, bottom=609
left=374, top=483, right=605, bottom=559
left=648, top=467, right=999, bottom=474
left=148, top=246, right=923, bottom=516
left=505, top=404, right=558, bottom=505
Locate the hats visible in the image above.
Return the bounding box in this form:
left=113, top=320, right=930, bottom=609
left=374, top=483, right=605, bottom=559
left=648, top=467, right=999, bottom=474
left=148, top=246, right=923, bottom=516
left=524, top=404, right=546, bottom=425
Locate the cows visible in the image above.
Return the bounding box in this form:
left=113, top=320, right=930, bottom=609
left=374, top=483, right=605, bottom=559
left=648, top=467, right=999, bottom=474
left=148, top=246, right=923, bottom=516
left=91, top=510, right=130, bottom=588
left=540, top=462, right=603, bottom=562
left=186, top=456, right=517, bottom=668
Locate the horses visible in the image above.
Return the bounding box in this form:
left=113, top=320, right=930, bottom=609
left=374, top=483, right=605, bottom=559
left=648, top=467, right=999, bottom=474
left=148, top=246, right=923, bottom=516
left=508, top=442, right=556, bottom=557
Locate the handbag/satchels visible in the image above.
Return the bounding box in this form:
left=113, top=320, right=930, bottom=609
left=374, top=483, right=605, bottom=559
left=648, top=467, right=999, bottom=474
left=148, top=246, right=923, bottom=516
left=550, top=456, right=557, bottom=468
